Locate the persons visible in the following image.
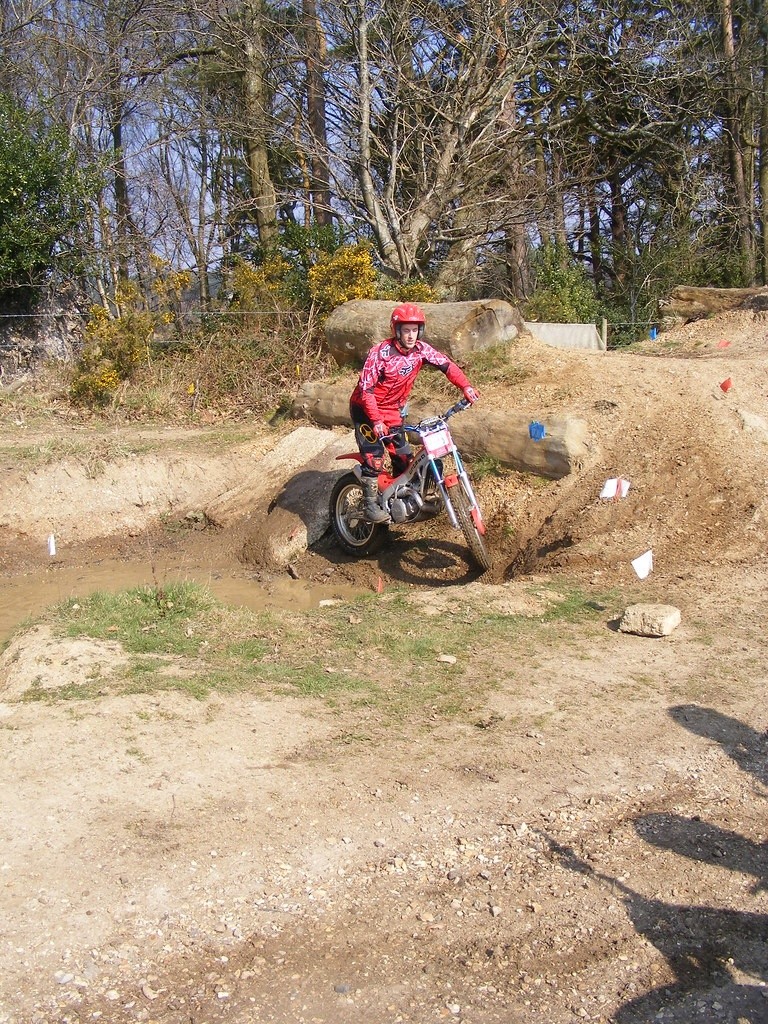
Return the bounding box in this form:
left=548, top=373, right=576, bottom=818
left=349, top=303, right=480, bottom=524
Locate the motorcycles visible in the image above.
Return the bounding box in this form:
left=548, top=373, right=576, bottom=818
left=328, top=394, right=492, bottom=572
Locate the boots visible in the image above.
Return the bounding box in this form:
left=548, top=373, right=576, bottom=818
left=361, top=476, right=390, bottom=523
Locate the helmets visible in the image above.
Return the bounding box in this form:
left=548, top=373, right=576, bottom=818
left=391, top=303, right=426, bottom=340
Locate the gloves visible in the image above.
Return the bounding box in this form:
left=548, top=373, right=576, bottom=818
left=373, top=420, right=388, bottom=437
left=464, top=386, right=480, bottom=405
